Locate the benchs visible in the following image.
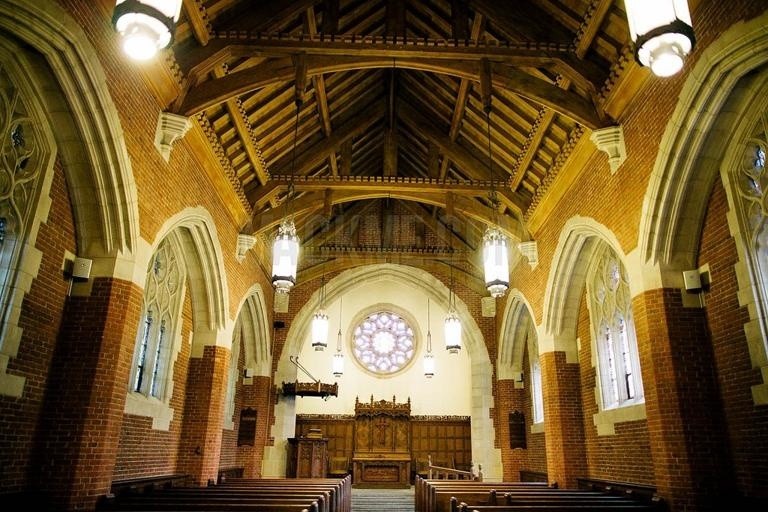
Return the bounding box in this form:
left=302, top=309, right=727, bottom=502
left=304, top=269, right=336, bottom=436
left=95, top=474, right=352, bottom=512
left=415, top=475, right=669, bottom=512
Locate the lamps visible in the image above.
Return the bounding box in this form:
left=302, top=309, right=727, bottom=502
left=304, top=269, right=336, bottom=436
left=271, top=100, right=344, bottom=378
left=424, top=108, right=509, bottom=378
left=625, top=1, right=697, bottom=78
left=112, top=0, right=184, bottom=62
